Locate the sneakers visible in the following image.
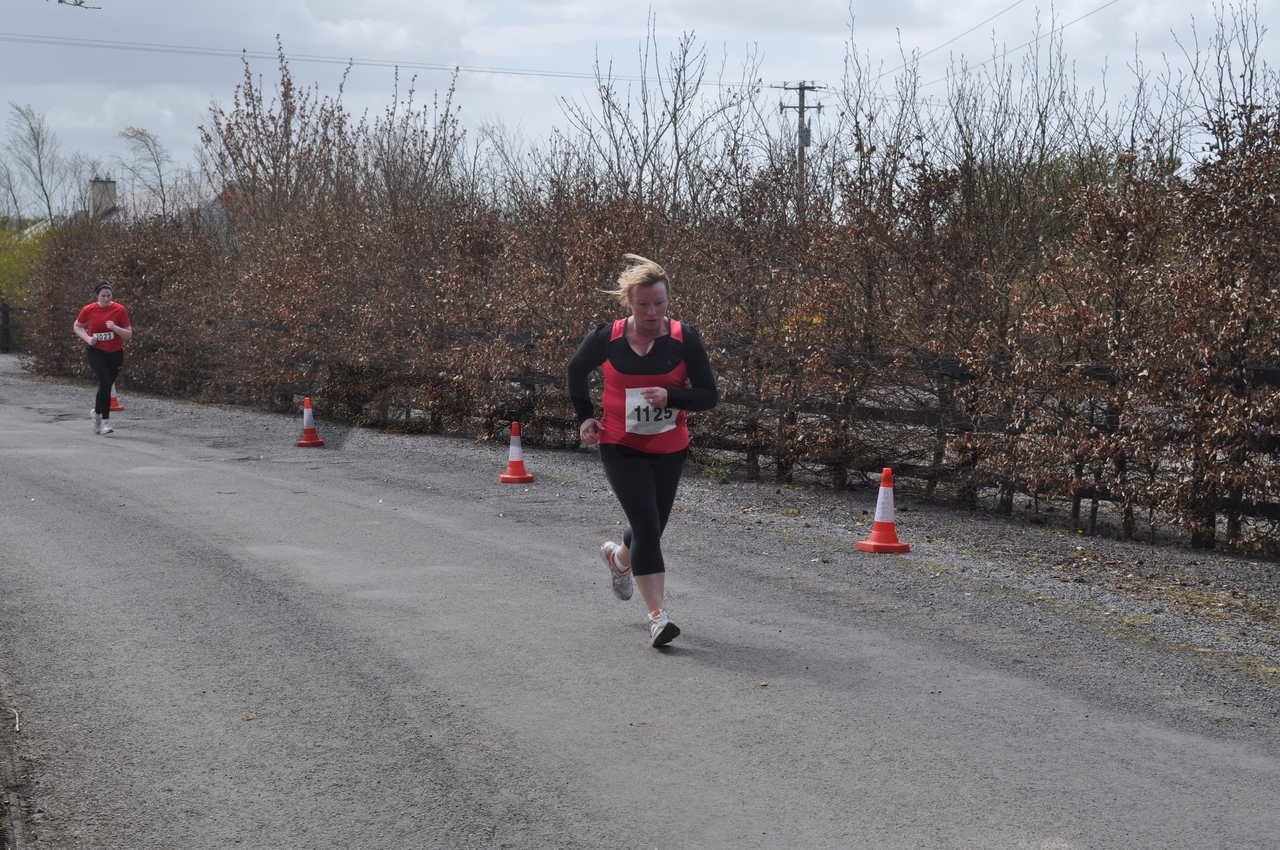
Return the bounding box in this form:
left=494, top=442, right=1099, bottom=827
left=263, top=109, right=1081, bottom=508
left=647, top=607, right=680, bottom=648
left=90, top=408, right=103, bottom=434
left=599, top=541, right=633, bottom=600
left=102, top=418, right=113, bottom=434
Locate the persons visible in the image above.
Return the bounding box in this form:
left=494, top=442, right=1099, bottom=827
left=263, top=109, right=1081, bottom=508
left=74, top=281, right=133, bottom=435
left=567, top=253, right=719, bottom=646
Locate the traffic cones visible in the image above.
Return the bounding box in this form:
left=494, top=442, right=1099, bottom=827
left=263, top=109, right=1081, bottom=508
left=296, top=397, right=324, bottom=447
left=499, top=421, right=534, bottom=484
left=110, top=382, right=124, bottom=411
left=856, top=467, right=911, bottom=554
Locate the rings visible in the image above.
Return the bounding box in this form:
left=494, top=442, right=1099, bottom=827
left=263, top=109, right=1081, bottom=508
left=650, top=399, right=653, bottom=403
